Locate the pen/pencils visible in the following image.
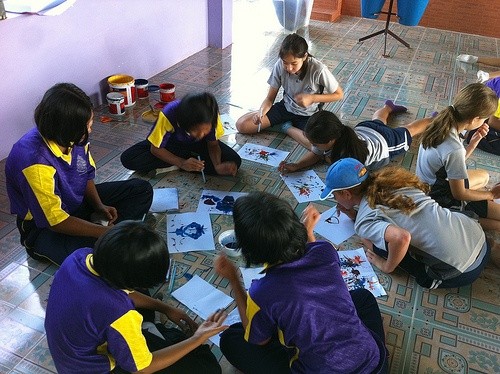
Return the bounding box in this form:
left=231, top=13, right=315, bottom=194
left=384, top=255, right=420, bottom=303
left=197, top=156, right=206, bottom=184
left=258, top=107, right=263, bottom=132
left=165, top=259, right=177, bottom=294
left=141, top=213, right=146, bottom=222
left=275, top=161, right=293, bottom=173
left=328, top=205, right=341, bottom=220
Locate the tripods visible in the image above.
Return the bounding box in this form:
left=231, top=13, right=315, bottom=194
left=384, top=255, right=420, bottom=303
left=358, top=0, right=410, bottom=56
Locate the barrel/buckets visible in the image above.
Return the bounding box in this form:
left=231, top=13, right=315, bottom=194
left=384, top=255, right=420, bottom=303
left=106, top=92, right=125, bottom=115
left=108, top=75, right=136, bottom=107
left=159, top=84, right=176, bottom=103
left=134, top=79, right=149, bottom=99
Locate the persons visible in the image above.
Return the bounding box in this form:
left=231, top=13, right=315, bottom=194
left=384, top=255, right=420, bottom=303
left=5, top=83, right=154, bottom=267
left=414, top=82, right=500, bottom=233
left=120, top=92, right=242, bottom=176
left=235, top=33, right=344, bottom=150
left=456, top=54, right=500, bottom=83
left=213, top=190, right=390, bottom=374
left=466, top=77, right=500, bottom=155
left=319, top=157, right=500, bottom=289
left=44, top=219, right=229, bottom=374
left=278, top=100, right=439, bottom=176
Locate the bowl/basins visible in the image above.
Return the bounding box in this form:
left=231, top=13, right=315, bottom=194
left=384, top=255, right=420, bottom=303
left=219, top=229, right=241, bottom=256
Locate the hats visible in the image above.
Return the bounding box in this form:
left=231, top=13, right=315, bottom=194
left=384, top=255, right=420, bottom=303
left=319, top=158, right=369, bottom=201
